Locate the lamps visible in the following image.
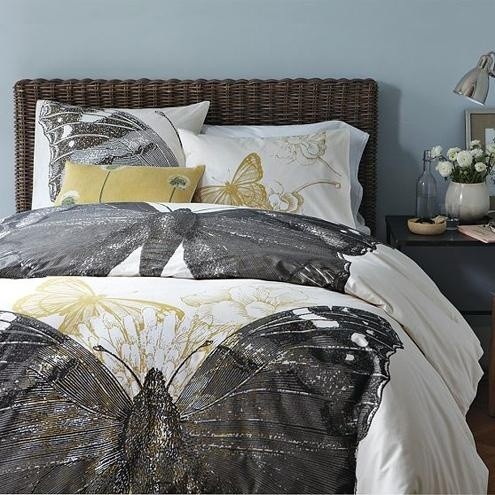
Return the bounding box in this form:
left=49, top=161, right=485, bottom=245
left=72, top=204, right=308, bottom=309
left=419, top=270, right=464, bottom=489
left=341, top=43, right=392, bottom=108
left=453, top=51, right=495, bottom=106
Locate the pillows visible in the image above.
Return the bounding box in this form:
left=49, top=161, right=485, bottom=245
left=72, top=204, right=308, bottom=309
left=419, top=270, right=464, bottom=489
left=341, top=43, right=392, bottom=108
left=204, top=118, right=369, bottom=226
left=177, top=125, right=358, bottom=228
left=33, top=99, right=210, bottom=210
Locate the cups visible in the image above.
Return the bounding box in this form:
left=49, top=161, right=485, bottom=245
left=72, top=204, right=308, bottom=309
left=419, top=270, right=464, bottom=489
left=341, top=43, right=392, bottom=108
left=445, top=207, right=459, bottom=232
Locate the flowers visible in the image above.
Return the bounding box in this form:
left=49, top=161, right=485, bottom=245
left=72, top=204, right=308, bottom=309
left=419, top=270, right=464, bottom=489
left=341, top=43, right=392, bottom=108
left=429, top=140, right=495, bottom=183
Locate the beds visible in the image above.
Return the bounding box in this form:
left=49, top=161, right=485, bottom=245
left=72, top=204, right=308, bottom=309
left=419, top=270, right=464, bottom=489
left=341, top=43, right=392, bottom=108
left=0, top=78, right=489, bottom=495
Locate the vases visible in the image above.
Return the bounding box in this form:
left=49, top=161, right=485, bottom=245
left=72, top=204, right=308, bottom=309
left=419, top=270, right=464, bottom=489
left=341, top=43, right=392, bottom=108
left=445, top=181, right=490, bottom=224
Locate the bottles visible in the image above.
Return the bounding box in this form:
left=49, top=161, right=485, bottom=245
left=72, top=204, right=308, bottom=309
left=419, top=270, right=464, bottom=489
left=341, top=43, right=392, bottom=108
left=417, top=150, right=437, bottom=217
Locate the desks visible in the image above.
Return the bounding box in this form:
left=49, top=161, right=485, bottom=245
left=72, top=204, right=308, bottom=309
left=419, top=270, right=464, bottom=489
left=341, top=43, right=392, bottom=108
left=385, top=215, right=495, bottom=316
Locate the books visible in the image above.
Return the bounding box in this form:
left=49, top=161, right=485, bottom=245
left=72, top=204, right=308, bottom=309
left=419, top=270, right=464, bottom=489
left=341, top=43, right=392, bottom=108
left=457, top=223, right=495, bottom=244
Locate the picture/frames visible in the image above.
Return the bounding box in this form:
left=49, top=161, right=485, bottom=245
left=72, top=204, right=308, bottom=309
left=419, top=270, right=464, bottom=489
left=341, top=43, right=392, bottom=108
left=465, top=109, right=495, bottom=213
